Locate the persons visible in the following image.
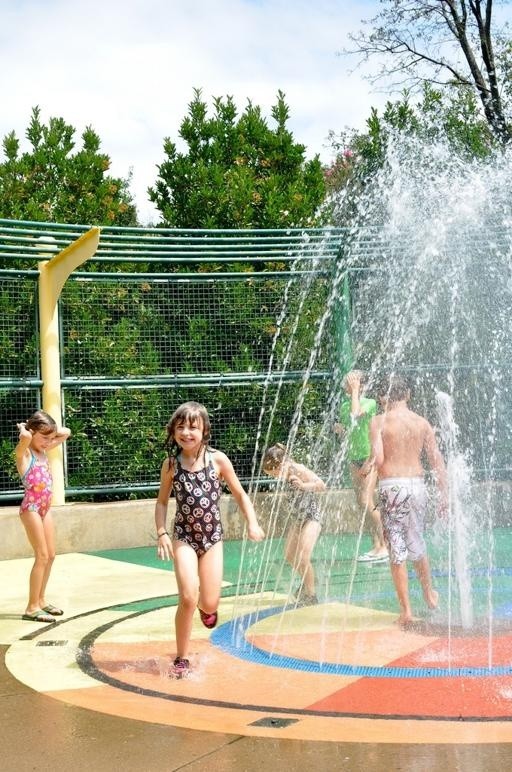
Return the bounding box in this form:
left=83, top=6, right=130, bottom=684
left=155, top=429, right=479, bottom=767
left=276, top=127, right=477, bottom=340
left=151, top=399, right=265, bottom=676
left=360, top=377, right=449, bottom=619
left=332, top=370, right=389, bottom=563
left=14, top=410, right=73, bottom=626
left=259, top=443, right=328, bottom=611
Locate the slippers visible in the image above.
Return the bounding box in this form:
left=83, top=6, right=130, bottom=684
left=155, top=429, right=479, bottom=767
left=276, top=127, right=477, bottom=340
left=21, top=609, right=56, bottom=623
left=42, top=604, right=64, bottom=616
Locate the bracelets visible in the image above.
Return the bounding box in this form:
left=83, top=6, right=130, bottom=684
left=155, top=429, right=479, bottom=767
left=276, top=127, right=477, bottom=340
left=157, top=530, right=170, bottom=540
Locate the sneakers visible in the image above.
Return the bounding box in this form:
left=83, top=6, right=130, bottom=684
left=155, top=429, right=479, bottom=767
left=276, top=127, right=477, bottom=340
left=195, top=604, right=219, bottom=629
left=168, top=656, right=191, bottom=678
left=294, top=593, right=319, bottom=609
left=357, top=547, right=390, bottom=564
left=294, top=583, right=305, bottom=597
